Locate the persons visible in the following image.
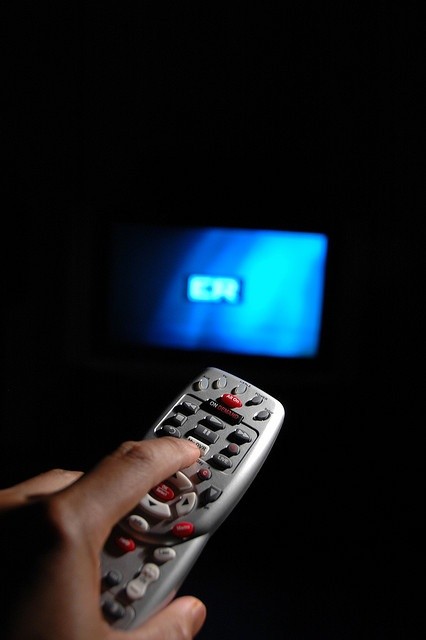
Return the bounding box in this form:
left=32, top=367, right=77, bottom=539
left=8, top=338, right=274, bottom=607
left=0, top=436, right=207, bottom=639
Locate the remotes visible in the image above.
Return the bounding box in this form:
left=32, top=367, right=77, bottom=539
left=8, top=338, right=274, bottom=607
left=33, top=367, right=286, bottom=618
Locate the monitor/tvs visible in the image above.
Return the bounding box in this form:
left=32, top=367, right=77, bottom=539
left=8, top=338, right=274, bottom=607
left=92, top=218, right=328, bottom=364
